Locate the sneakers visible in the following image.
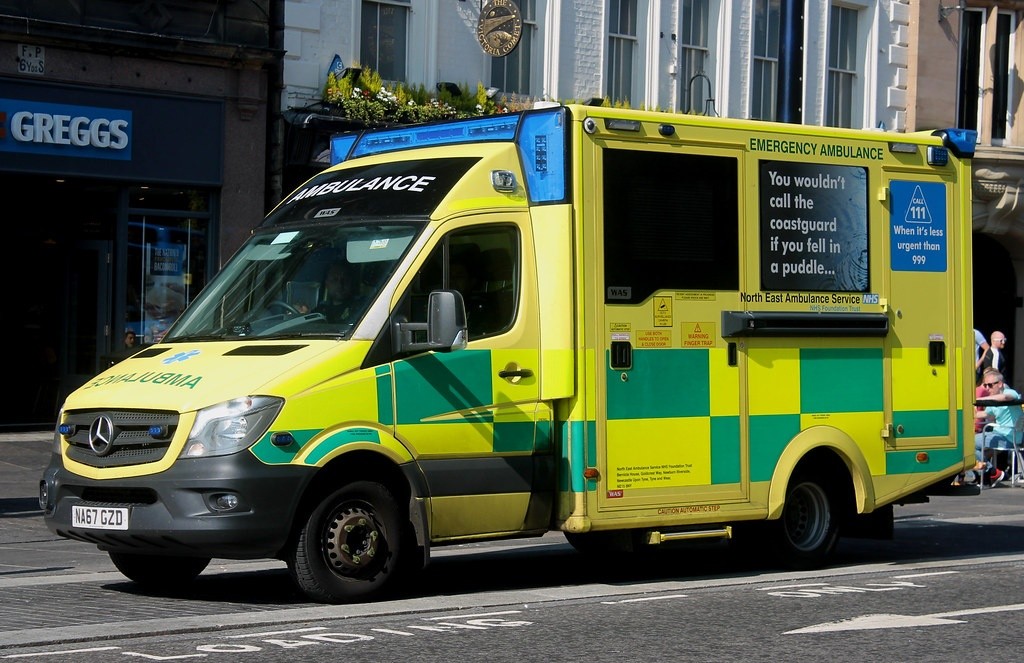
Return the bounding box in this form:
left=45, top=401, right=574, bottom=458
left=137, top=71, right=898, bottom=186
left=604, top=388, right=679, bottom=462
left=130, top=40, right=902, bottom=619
left=989, top=468, right=1005, bottom=488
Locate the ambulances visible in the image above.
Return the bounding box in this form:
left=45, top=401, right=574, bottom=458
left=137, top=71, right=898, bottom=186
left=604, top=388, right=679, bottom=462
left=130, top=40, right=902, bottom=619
left=38, top=99, right=987, bottom=605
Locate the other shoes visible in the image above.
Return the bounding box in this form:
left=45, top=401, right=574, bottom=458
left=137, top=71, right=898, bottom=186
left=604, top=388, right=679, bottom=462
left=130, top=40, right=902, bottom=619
left=951, top=481, right=966, bottom=485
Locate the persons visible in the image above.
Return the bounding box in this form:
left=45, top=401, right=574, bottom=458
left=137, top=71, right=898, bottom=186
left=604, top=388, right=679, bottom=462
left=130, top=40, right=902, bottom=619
left=123, top=331, right=138, bottom=347
left=294, top=265, right=361, bottom=324
left=416, top=257, right=496, bottom=334
left=952, top=328, right=1024, bottom=487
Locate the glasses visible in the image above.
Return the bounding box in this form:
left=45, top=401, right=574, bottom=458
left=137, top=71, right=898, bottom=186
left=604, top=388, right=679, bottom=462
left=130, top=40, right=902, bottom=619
left=993, top=338, right=1006, bottom=342
left=983, top=381, right=999, bottom=388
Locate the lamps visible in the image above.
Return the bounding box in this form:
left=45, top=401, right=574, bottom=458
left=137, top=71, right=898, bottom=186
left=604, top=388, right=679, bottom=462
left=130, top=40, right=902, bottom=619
left=477, top=86, right=500, bottom=100
left=583, top=98, right=605, bottom=106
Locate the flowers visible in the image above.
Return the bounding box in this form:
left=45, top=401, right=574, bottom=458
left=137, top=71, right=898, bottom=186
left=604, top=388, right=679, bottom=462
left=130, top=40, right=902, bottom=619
left=326, top=61, right=508, bottom=118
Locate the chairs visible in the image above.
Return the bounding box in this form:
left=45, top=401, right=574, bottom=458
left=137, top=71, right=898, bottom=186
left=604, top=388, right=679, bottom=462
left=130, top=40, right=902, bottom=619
left=980, top=422, right=1024, bottom=491
